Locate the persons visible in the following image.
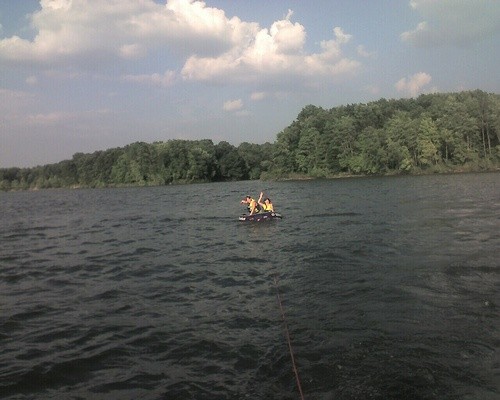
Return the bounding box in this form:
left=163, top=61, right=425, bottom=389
left=240, top=195, right=260, bottom=216
left=257, top=192, right=274, bottom=212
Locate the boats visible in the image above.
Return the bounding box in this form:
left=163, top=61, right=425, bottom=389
left=239, top=213, right=283, bottom=222
